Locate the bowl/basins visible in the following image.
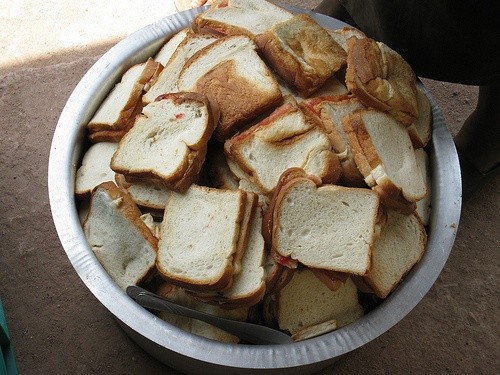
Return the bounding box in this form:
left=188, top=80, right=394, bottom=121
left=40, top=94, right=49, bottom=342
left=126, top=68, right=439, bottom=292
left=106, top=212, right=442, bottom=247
left=46, top=5, right=463, bottom=369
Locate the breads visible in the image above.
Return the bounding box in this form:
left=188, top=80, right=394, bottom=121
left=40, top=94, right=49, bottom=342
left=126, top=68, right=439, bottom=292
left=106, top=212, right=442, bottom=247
left=75, top=0, right=433, bottom=342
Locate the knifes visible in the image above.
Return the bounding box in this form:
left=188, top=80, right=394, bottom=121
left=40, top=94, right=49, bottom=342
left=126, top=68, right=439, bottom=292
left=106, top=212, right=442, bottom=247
left=134, top=293, right=294, bottom=344
